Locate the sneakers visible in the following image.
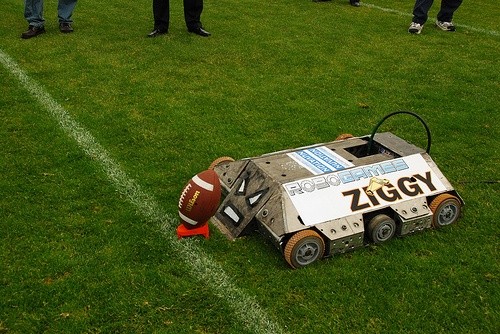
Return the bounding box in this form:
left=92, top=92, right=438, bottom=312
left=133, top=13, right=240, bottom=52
left=435, top=19, right=456, bottom=32
left=408, top=21, right=424, bottom=34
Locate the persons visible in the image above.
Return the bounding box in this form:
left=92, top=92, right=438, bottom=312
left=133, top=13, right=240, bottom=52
left=312, top=0, right=360, bottom=7
left=21, top=0, right=78, bottom=39
left=408, top=0, right=463, bottom=34
left=147, top=0, right=211, bottom=37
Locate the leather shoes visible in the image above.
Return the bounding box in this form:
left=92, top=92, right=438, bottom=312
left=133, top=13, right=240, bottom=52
left=22, top=24, right=47, bottom=39
left=59, top=22, right=75, bottom=34
left=147, top=29, right=168, bottom=37
left=188, top=26, right=211, bottom=37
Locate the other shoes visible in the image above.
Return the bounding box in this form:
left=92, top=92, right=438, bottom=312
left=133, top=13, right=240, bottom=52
left=350, top=0, right=360, bottom=7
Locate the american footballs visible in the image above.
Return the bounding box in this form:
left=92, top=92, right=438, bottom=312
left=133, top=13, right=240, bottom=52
left=178, top=170, right=221, bottom=230
